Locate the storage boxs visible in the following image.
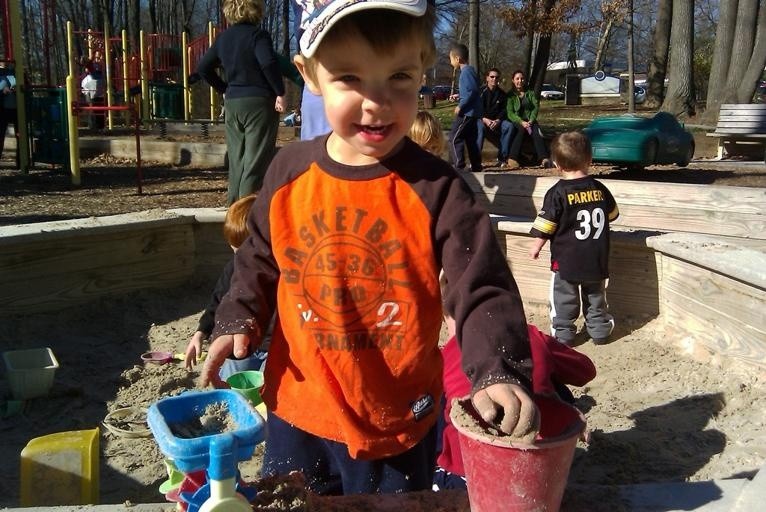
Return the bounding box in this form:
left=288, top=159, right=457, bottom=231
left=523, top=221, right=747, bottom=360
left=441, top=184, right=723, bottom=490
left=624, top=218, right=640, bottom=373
left=20, top=427, right=99, bottom=509
left=3, top=347, right=59, bottom=400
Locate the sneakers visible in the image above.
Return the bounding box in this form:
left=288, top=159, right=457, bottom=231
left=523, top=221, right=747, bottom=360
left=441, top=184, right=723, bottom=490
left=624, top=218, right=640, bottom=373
left=501, top=160, right=509, bottom=168
left=555, top=336, right=576, bottom=347
left=467, top=166, right=482, bottom=172
left=592, top=336, right=610, bottom=346
left=541, top=158, right=552, bottom=169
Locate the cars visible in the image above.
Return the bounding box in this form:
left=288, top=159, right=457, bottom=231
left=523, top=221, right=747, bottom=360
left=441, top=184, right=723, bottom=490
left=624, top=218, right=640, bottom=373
left=419, top=85, right=458, bottom=100
left=540, top=84, right=565, bottom=101
left=758, top=80, right=766, bottom=94
left=635, top=84, right=648, bottom=103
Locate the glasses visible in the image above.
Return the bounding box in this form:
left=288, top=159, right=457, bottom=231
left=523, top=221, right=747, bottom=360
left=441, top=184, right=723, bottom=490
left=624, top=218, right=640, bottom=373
left=489, top=75, right=500, bottom=79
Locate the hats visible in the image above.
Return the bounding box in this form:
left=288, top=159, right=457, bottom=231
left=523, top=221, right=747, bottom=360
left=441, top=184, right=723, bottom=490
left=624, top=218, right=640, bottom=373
left=287, top=0, right=429, bottom=62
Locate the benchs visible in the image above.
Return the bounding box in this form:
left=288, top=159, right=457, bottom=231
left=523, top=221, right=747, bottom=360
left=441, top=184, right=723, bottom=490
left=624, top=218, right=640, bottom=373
left=706, top=104, right=766, bottom=161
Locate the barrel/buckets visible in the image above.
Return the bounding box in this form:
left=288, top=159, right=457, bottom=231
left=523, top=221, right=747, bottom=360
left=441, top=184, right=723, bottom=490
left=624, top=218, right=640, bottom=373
left=225, top=370, right=265, bottom=409
left=448, top=391, right=588, bottom=512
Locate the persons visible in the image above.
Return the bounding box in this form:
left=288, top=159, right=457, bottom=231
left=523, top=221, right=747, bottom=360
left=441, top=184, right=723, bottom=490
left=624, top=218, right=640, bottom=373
left=182, top=193, right=275, bottom=381
left=0, top=51, right=109, bottom=170
left=403, top=110, right=451, bottom=168
left=527, top=129, right=621, bottom=347
left=473, top=67, right=516, bottom=169
left=504, top=70, right=552, bottom=171
left=202, top=1, right=545, bottom=501
left=447, top=43, right=486, bottom=174
left=435, top=306, right=596, bottom=492
left=196, top=1, right=292, bottom=208
